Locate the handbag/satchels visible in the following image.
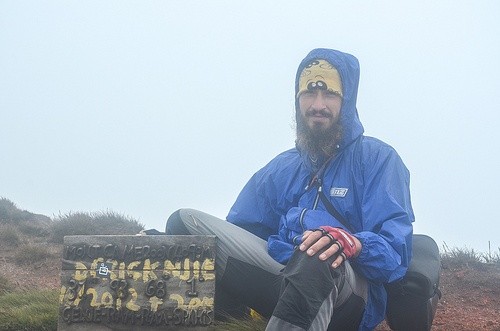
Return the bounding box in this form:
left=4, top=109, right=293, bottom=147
left=383, top=234, right=443, bottom=331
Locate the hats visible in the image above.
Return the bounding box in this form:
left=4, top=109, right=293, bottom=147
left=296, top=60, right=343, bottom=99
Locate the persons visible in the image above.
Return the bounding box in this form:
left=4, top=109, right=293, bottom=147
left=160, top=48, right=416, bottom=331
